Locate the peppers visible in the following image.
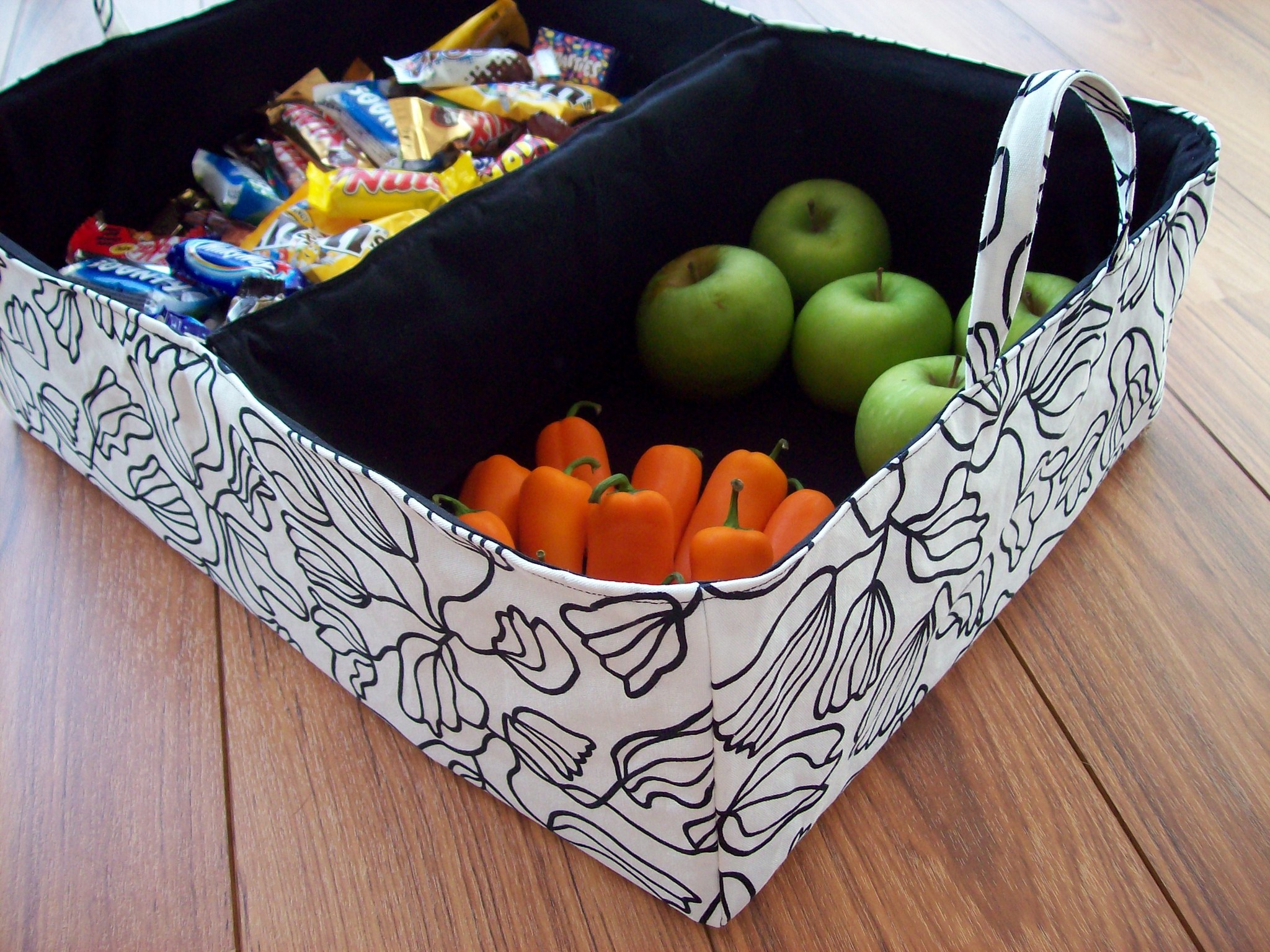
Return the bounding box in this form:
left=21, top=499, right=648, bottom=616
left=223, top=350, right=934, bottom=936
left=434, top=398, right=836, bottom=585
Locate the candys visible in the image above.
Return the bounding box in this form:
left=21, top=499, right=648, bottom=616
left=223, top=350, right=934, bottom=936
left=57, top=0, right=624, bottom=328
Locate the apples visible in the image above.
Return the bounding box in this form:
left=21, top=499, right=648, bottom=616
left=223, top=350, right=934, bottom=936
left=749, top=179, right=890, bottom=312
left=854, top=354, right=965, bottom=476
left=951, top=272, right=1080, bottom=356
left=634, top=246, right=793, bottom=395
left=792, top=266, right=955, bottom=417
